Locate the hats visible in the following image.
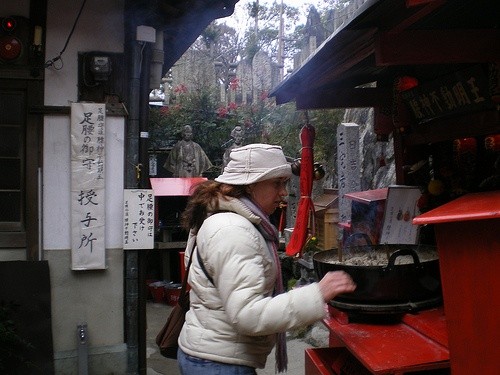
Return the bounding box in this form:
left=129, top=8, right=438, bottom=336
left=214, top=144, right=291, bottom=185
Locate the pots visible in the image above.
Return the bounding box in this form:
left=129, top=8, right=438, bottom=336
left=312, top=231, right=443, bottom=303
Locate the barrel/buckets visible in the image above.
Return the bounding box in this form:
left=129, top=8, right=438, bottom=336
left=146, top=279, right=184, bottom=306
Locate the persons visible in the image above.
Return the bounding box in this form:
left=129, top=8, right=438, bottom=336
left=163, top=125, right=214, bottom=177
left=222, top=126, right=245, bottom=174
left=177, top=143, right=357, bottom=375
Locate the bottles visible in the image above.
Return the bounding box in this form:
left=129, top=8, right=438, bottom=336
left=428, top=155, right=444, bottom=198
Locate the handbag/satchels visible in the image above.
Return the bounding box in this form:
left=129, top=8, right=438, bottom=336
left=156, top=292, right=190, bottom=360
